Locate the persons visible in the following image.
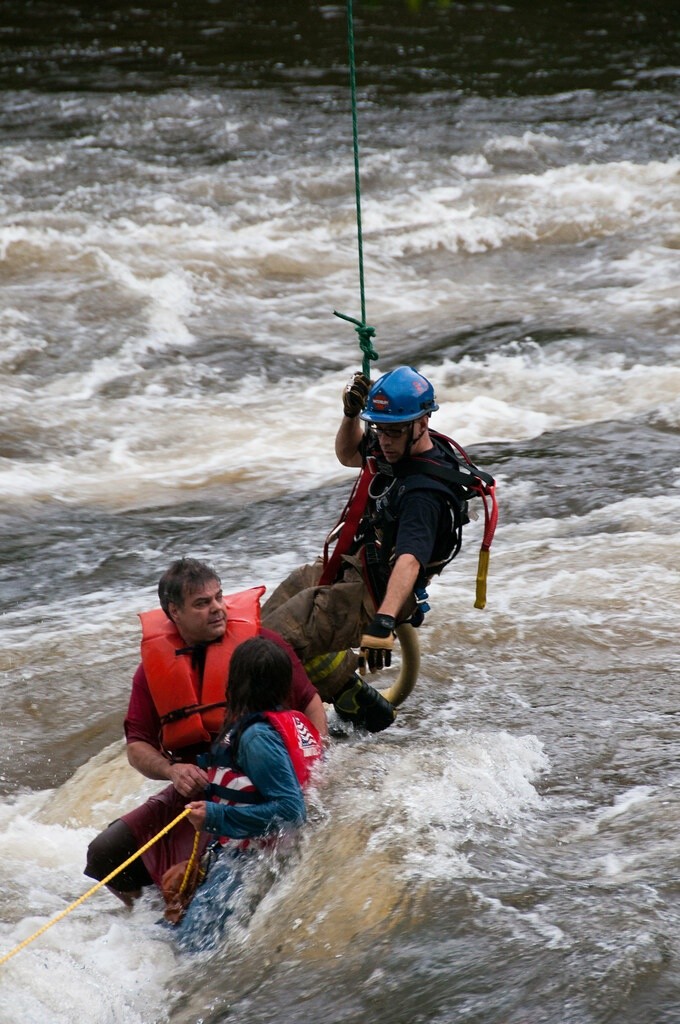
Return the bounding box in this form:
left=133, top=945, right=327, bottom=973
left=83, top=558, right=332, bottom=907
left=165, top=638, right=324, bottom=953
left=259, top=364, right=470, bottom=732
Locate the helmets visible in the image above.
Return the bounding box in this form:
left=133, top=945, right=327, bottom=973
left=358, top=365, right=440, bottom=425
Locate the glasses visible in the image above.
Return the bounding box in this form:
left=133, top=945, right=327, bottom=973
left=369, top=423, right=412, bottom=438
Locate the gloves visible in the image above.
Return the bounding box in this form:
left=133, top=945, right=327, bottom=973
left=358, top=613, right=398, bottom=676
left=342, top=370, right=371, bottom=418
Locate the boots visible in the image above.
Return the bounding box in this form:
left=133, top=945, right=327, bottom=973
left=328, top=673, right=397, bottom=740
